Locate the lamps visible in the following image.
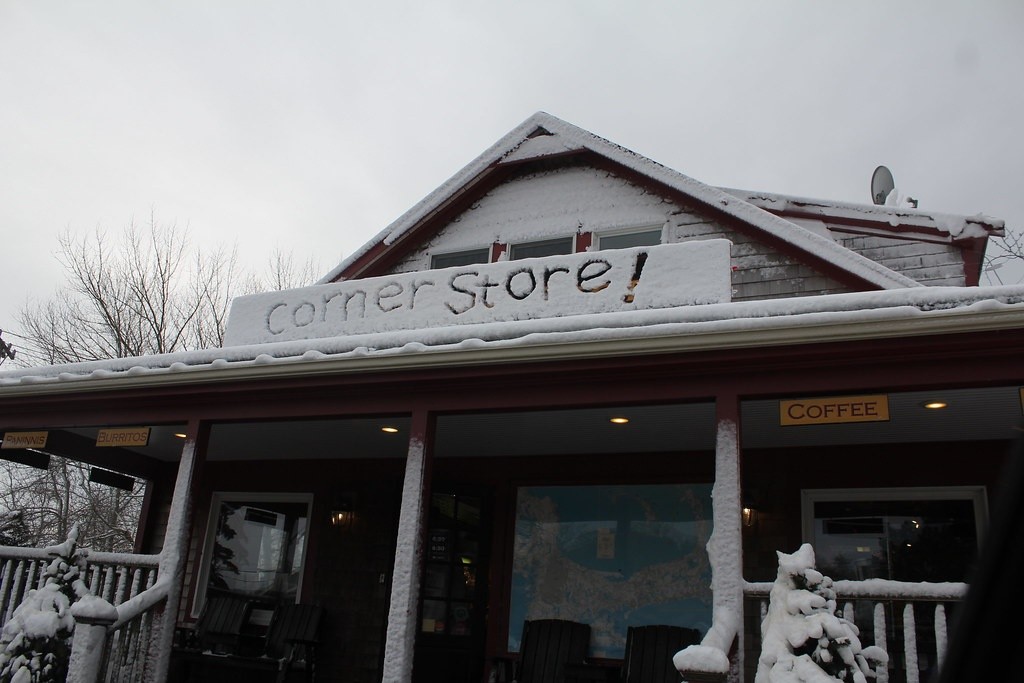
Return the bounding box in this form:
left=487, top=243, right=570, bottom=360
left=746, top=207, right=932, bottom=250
left=742, top=493, right=760, bottom=529
left=330, top=498, right=362, bottom=536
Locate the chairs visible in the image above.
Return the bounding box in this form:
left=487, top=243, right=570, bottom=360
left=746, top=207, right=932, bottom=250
left=173, top=598, right=328, bottom=683
left=483, top=620, right=699, bottom=683
